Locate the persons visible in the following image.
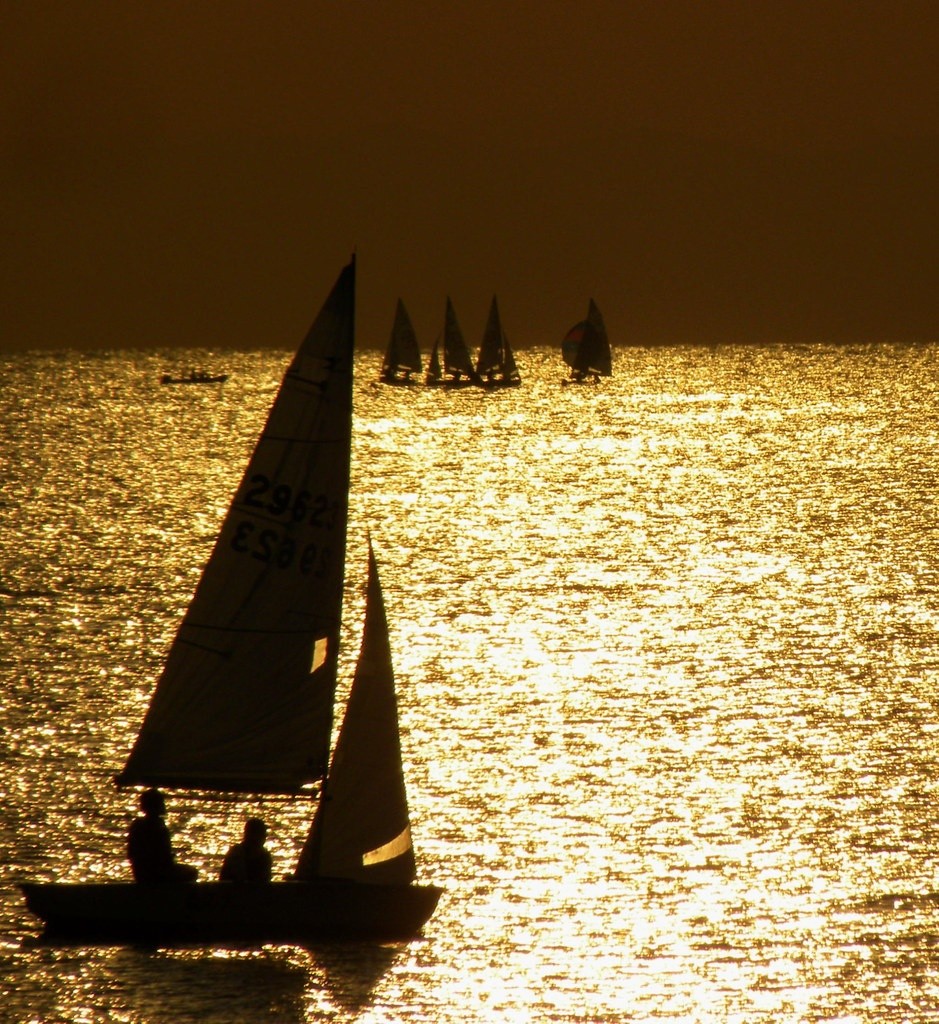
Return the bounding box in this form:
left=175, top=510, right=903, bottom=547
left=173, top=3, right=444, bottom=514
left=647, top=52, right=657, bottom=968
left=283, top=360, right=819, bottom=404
left=127, top=789, right=199, bottom=883
left=217, top=816, right=270, bottom=885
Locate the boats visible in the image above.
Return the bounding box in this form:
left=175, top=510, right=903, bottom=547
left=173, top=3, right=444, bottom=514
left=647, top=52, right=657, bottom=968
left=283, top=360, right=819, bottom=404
left=160, top=372, right=229, bottom=384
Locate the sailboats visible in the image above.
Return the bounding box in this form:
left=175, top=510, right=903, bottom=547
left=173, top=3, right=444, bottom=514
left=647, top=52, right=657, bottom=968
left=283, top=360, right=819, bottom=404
left=559, top=295, right=614, bottom=385
left=379, top=290, right=522, bottom=388
left=11, top=243, right=450, bottom=954
